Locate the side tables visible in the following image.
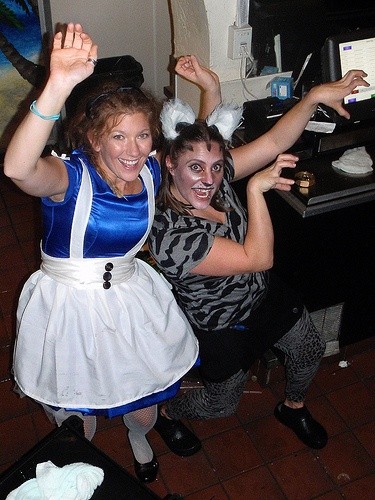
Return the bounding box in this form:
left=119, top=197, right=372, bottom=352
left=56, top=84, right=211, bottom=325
left=0, top=414, right=185, bottom=500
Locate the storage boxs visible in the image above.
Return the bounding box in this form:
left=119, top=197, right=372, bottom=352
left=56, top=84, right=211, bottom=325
left=271, top=76, right=293, bottom=99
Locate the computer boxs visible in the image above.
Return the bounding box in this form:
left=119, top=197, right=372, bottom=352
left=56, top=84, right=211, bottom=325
left=304, top=111, right=375, bottom=152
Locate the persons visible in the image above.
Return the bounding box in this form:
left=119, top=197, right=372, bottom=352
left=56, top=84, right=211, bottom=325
left=3, top=23, right=222, bottom=484
left=146, top=70, right=371, bottom=458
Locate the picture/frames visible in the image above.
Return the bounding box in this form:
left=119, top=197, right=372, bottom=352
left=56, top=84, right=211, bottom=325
left=0, top=0, right=67, bottom=165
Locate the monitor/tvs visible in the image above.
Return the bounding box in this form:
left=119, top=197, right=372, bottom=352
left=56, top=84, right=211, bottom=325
left=321, top=29, right=375, bottom=125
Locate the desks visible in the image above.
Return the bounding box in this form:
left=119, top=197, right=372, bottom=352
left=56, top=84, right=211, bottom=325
left=217, top=129, right=375, bottom=350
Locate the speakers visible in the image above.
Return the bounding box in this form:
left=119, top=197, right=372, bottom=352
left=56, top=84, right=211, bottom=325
left=243, top=97, right=303, bottom=159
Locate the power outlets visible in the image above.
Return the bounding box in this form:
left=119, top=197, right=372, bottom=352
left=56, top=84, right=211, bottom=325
left=228, top=24, right=253, bottom=60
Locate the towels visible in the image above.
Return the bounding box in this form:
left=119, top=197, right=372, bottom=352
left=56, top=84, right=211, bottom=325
left=5, top=461, right=105, bottom=500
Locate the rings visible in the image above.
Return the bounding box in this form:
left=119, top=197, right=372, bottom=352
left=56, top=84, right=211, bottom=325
left=64, top=45, right=71, bottom=48
left=87, top=58, right=98, bottom=66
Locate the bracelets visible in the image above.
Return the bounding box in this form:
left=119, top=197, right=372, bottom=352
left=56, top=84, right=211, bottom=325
left=29, top=100, right=60, bottom=121
